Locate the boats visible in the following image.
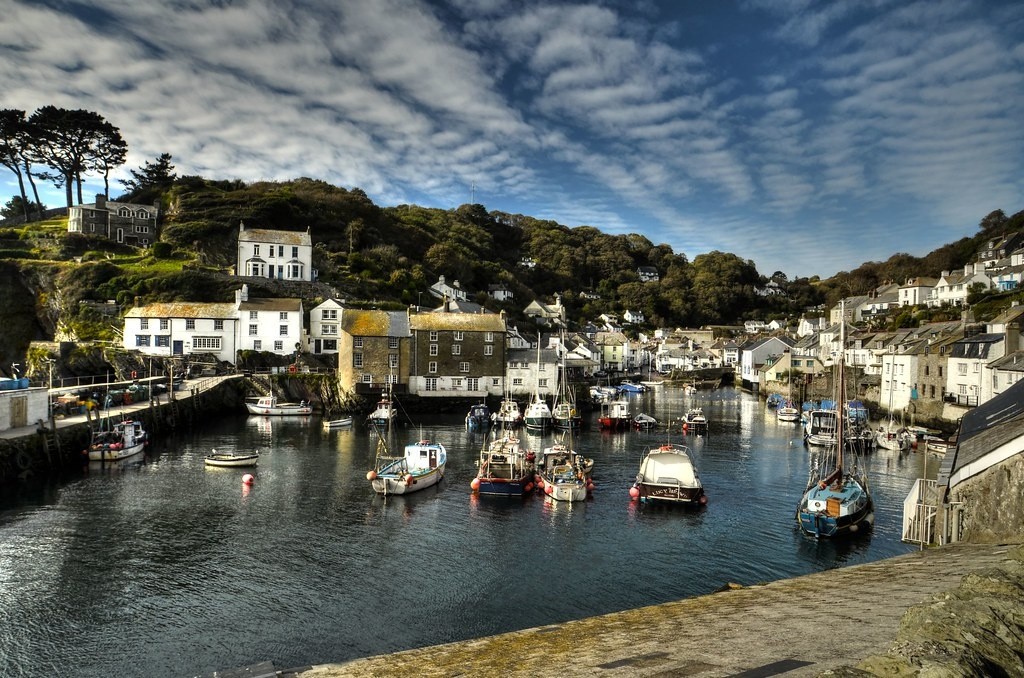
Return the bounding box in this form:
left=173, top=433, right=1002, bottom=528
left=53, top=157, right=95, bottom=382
left=365, top=423, right=448, bottom=496
left=534, top=417, right=595, bottom=503
left=588, top=383, right=620, bottom=405
left=684, top=385, right=699, bottom=394
left=843, top=414, right=874, bottom=445
left=538, top=455, right=594, bottom=474
left=599, top=397, right=632, bottom=431
left=464, top=400, right=495, bottom=431
left=924, top=433, right=946, bottom=442
left=204, top=447, right=262, bottom=466
left=487, top=399, right=524, bottom=430
left=805, top=408, right=842, bottom=446
left=242, top=376, right=314, bottom=415
left=366, top=362, right=397, bottom=427
left=924, top=439, right=954, bottom=455
left=86, top=448, right=146, bottom=474
left=632, top=411, right=656, bottom=430
left=630, top=442, right=708, bottom=507
left=682, top=406, right=708, bottom=435
left=614, top=379, right=649, bottom=394
left=470, top=393, right=538, bottom=497
left=639, top=381, right=666, bottom=385
left=907, top=425, right=943, bottom=437
left=83, top=416, right=148, bottom=460
left=776, top=405, right=800, bottom=421
left=244, top=415, right=312, bottom=438
left=322, top=415, right=354, bottom=428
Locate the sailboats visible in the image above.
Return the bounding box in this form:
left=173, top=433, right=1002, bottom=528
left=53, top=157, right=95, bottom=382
left=875, top=345, right=913, bottom=452
left=552, top=331, right=583, bottom=430
left=520, top=331, right=554, bottom=434
left=798, top=297, right=877, bottom=545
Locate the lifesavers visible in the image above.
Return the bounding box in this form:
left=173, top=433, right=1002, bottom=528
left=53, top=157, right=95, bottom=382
left=75, top=430, right=90, bottom=448
left=182, top=408, right=193, bottom=423
left=16, top=452, right=35, bottom=483
left=131, top=370, right=138, bottom=379
left=289, top=366, right=296, bottom=374
left=167, top=415, right=176, bottom=429
